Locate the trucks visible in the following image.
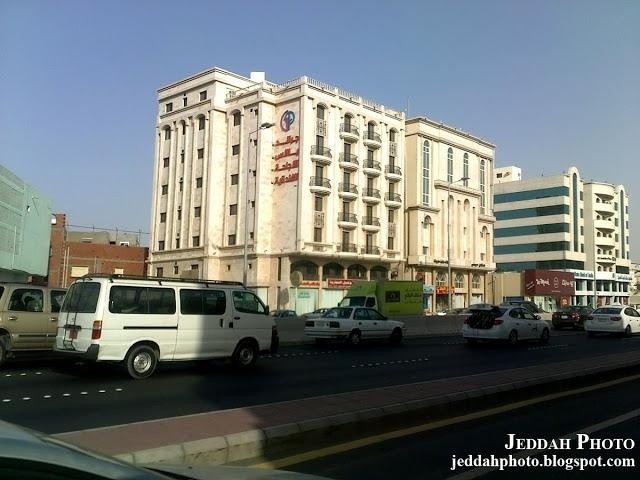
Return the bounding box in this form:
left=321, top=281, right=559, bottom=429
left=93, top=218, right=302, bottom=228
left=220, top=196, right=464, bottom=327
left=338, top=280, right=424, bottom=318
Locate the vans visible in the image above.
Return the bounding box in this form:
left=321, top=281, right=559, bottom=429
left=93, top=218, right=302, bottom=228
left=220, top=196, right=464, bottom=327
left=54, top=272, right=279, bottom=381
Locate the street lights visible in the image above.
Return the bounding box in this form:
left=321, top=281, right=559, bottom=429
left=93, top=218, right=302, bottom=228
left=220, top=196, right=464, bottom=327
left=447, top=177, right=471, bottom=313
left=243, top=123, right=274, bottom=290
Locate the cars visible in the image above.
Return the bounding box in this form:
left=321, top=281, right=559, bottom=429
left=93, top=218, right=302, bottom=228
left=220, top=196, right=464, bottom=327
left=462, top=306, right=550, bottom=345
left=0, top=282, right=68, bottom=370
left=552, top=306, right=640, bottom=336
left=303, top=306, right=409, bottom=346
left=450, top=301, right=545, bottom=313
left=270, top=308, right=330, bottom=319
left=0, top=418, right=330, bottom=480
